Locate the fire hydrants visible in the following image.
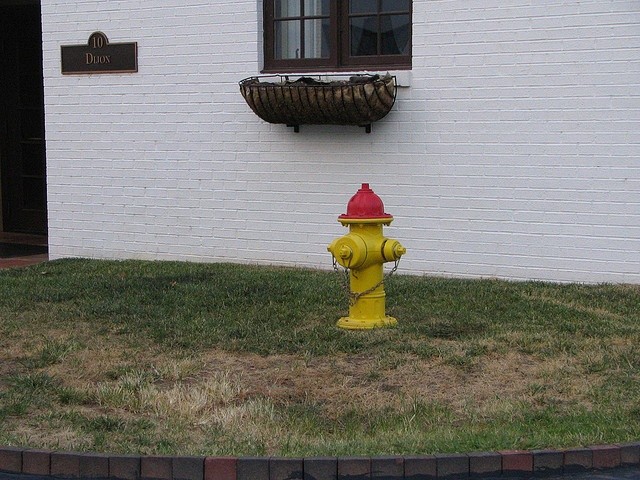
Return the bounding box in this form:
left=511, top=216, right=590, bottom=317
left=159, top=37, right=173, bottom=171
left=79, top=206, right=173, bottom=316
left=327, top=183, right=406, bottom=330
